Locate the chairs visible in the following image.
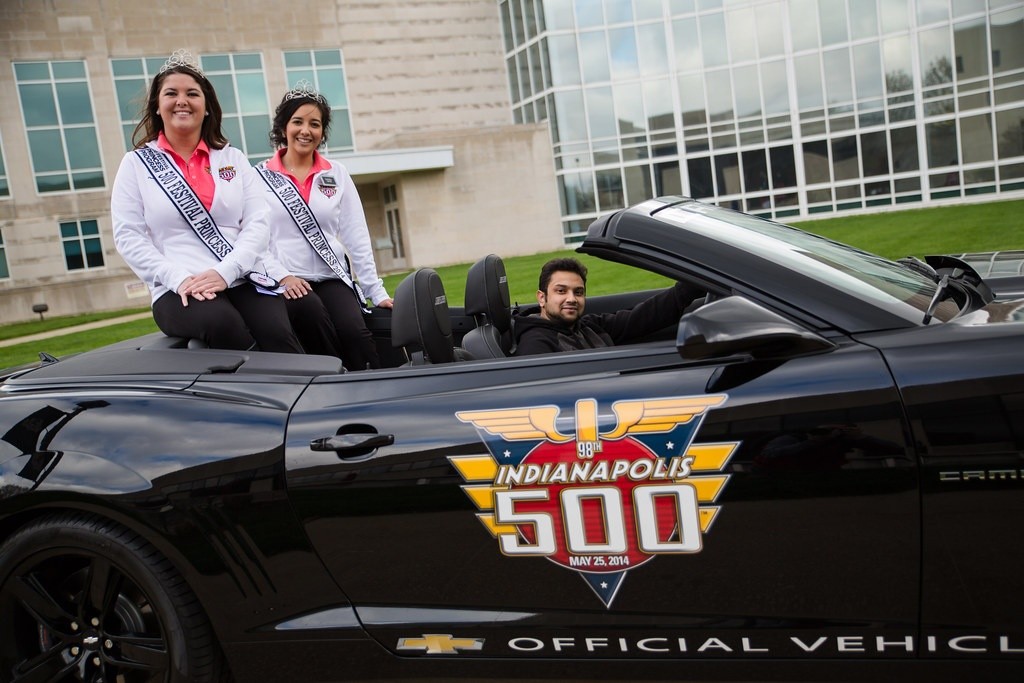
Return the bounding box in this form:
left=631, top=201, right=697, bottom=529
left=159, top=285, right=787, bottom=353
left=462, top=254, right=515, bottom=360
left=391, top=268, right=476, bottom=368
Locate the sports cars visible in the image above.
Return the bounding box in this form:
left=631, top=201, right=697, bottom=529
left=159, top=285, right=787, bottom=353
left=2, top=194, right=1022, bottom=677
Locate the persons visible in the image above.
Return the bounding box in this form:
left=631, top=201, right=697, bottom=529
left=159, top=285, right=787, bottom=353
left=252, top=90, right=394, bottom=371
left=513, top=257, right=706, bottom=353
left=111, top=62, right=303, bottom=353
